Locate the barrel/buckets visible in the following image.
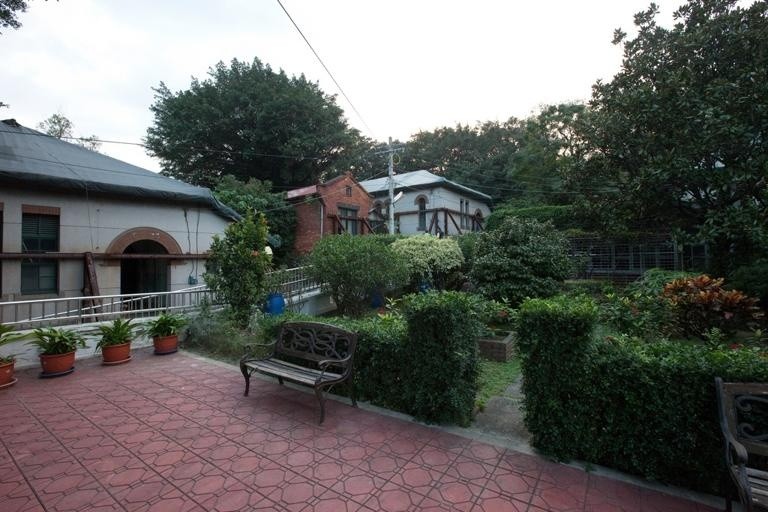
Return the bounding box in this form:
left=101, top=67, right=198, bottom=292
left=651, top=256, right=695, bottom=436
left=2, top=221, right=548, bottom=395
left=266, top=292, right=286, bottom=313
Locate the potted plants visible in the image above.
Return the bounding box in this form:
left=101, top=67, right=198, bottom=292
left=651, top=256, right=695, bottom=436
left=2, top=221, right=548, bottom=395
left=0, top=307, right=191, bottom=392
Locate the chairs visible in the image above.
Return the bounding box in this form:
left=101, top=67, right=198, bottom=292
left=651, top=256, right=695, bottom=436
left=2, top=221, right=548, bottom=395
left=713, top=375, right=768, bottom=512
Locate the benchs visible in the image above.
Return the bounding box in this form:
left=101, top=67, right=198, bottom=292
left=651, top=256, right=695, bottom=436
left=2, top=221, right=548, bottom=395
left=240, top=320, right=359, bottom=426
